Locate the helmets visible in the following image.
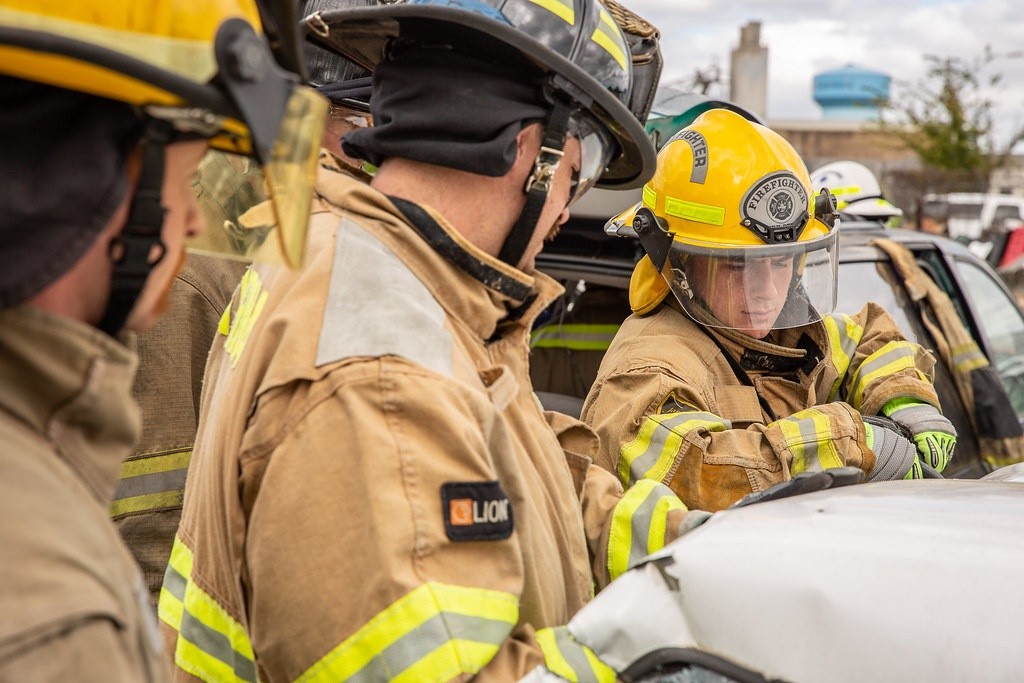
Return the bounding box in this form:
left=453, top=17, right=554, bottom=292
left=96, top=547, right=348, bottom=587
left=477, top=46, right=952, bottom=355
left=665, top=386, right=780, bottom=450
left=602, top=107, right=836, bottom=259
left=0, top=1, right=293, bottom=155
left=809, top=160, right=902, bottom=215
left=300, top=1, right=657, bottom=190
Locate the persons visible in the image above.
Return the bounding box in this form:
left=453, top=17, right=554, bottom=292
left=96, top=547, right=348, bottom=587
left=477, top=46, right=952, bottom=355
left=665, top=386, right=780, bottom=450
left=580, top=107, right=957, bottom=514
left=0, top=0, right=904, bottom=683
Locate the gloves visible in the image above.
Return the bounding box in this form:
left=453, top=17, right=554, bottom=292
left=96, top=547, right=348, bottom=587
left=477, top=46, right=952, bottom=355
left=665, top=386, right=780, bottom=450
left=861, top=422, right=924, bottom=482
left=881, top=396, right=958, bottom=472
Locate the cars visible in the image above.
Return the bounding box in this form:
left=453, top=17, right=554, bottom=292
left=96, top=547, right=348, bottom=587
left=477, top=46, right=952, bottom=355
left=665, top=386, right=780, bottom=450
left=802, top=191, right=1024, bottom=480
